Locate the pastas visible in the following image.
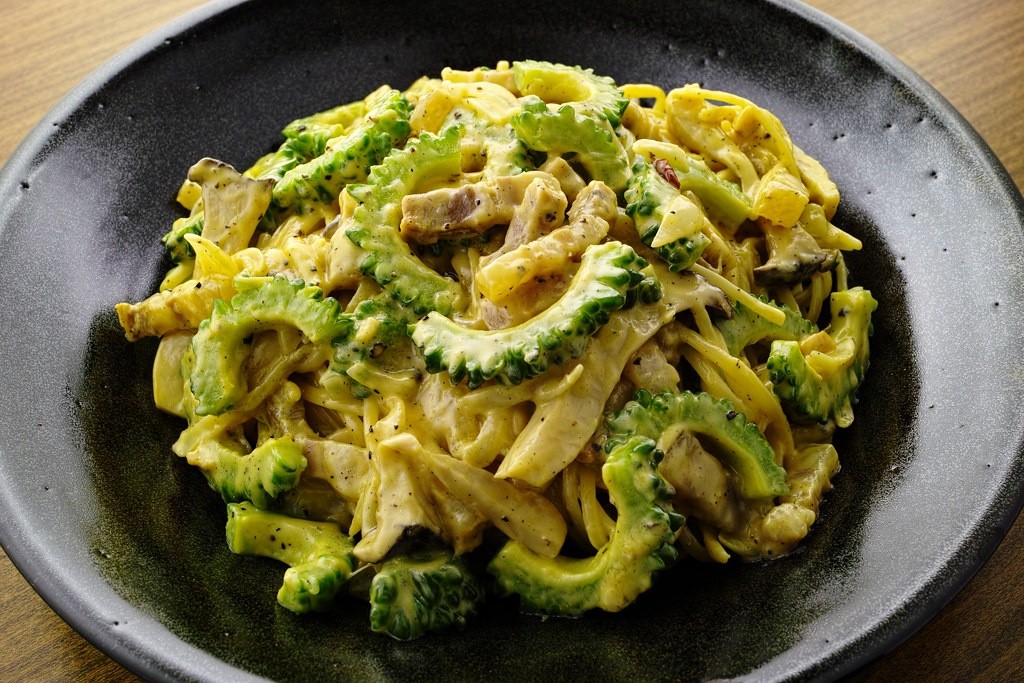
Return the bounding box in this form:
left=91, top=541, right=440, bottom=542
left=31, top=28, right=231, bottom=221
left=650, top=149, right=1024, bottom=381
left=165, top=59, right=848, bottom=569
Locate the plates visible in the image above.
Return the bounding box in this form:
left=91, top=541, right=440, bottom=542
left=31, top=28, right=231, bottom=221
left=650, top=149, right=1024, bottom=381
left=0, top=0, right=1024, bottom=683
